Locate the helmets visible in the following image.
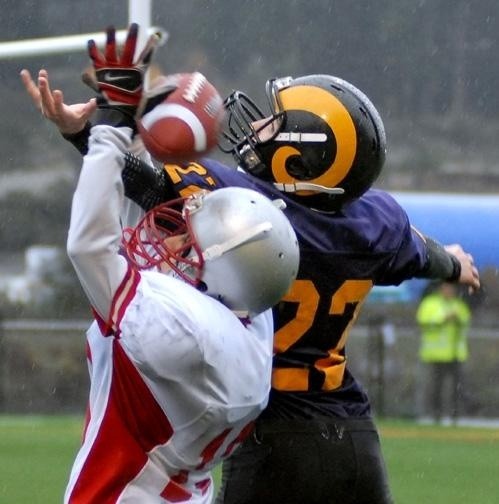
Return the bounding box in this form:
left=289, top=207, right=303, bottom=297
left=124, top=184, right=302, bottom=327
left=212, top=76, right=389, bottom=215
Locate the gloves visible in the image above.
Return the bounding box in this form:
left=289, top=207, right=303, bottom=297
left=80, top=19, right=167, bottom=111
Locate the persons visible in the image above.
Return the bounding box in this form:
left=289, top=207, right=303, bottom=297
left=415, top=277, right=472, bottom=425
left=19, top=68, right=481, bottom=504
left=62, top=21, right=302, bottom=504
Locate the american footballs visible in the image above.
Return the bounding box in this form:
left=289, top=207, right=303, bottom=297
left=138, top=71, right=224, bottom=165
